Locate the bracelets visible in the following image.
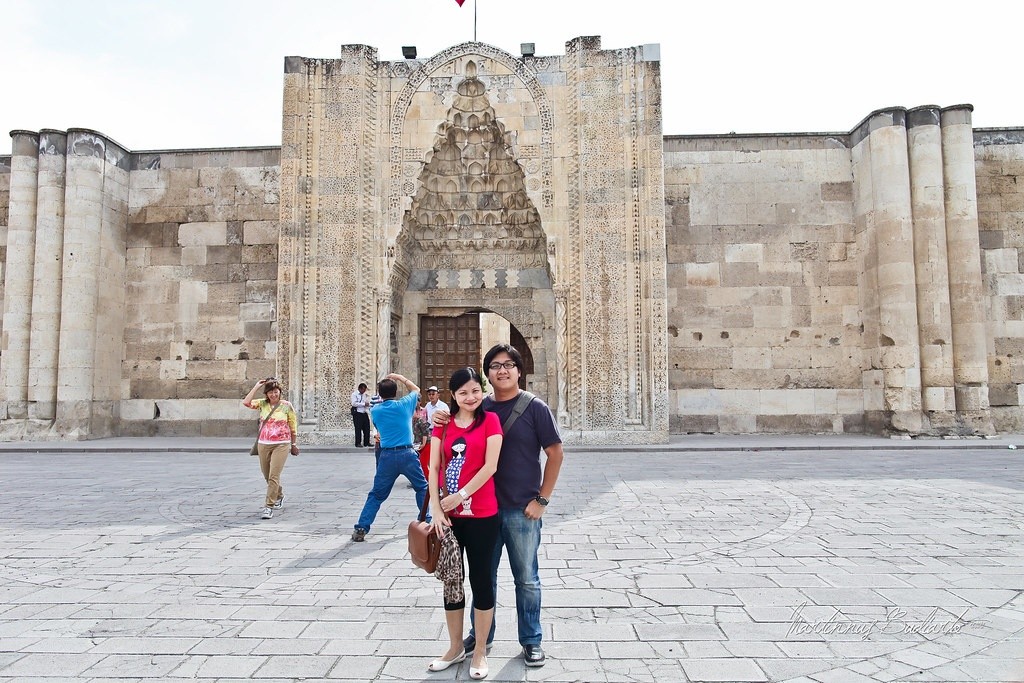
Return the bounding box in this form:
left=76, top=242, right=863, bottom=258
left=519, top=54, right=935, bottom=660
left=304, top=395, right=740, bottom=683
left=405, top=379, right=408, bottom=385
left=291, top=443, right=296, bottom=446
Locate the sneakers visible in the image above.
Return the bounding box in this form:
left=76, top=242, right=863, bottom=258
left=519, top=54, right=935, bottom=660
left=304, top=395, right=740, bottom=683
left=261, top=507, right=273, bottom=519
left=352, top=528, right=365, bottom=541
left=273, top=498, right=284, bottom=509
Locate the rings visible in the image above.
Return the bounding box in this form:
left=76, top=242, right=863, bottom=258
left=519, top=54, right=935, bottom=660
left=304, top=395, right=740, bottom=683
left=435, top=525, right=438, bottom=527
left=434, top=418, right=437, bottom=421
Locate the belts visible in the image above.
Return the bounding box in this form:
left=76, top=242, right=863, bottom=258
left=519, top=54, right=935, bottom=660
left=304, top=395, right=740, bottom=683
left=383, top=445, right=413, bottom=450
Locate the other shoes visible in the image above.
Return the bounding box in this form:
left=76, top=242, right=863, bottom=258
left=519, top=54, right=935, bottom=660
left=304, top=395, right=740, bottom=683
left=470, top=656, right=488, bottom=679
left=357, top=444, right=363, bottom=447
left=366, top=443, right=374, bottom=447
left=428, top=648, right=465, bottom=671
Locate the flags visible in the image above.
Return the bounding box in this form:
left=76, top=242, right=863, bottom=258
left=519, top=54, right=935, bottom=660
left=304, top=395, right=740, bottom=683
left=455, top=0, right=465, bottom=7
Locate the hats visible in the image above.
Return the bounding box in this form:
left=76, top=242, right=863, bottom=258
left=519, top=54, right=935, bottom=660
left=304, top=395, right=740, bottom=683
left=426, top=386, right=439, bottom=393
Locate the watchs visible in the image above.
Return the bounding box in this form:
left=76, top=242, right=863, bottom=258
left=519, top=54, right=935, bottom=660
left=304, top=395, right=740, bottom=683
left=535, top=494, right=549, bottom=507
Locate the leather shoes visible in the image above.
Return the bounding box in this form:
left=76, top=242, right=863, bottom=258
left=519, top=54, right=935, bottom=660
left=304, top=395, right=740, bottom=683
left=463, top=635, right=493, bottom=656
left=524, top=644, right=546, bottom=666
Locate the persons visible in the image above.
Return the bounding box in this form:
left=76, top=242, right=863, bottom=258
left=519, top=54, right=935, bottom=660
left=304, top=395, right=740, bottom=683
left=432, top=344, right=564, bottom=667
left=243, top=377, right=299, bottom=519
left=350, top=383, right=374, bottom=447
left=351, top=373, right=451, bottom=542
left=427, top=367, right=508, bottom=679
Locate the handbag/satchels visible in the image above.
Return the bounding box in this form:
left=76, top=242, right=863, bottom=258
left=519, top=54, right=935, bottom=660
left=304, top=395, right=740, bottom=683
left=409, top=521, right=441, bottom=573
left=250, top=442, right=259, bottom=455
left=350, top=406, right=357, bottom=415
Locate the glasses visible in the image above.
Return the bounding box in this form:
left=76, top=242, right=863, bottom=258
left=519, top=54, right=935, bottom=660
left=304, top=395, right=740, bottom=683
left=266, top=377, right=275, bottom=381
left=489, top=362, right=517, bottom=370
